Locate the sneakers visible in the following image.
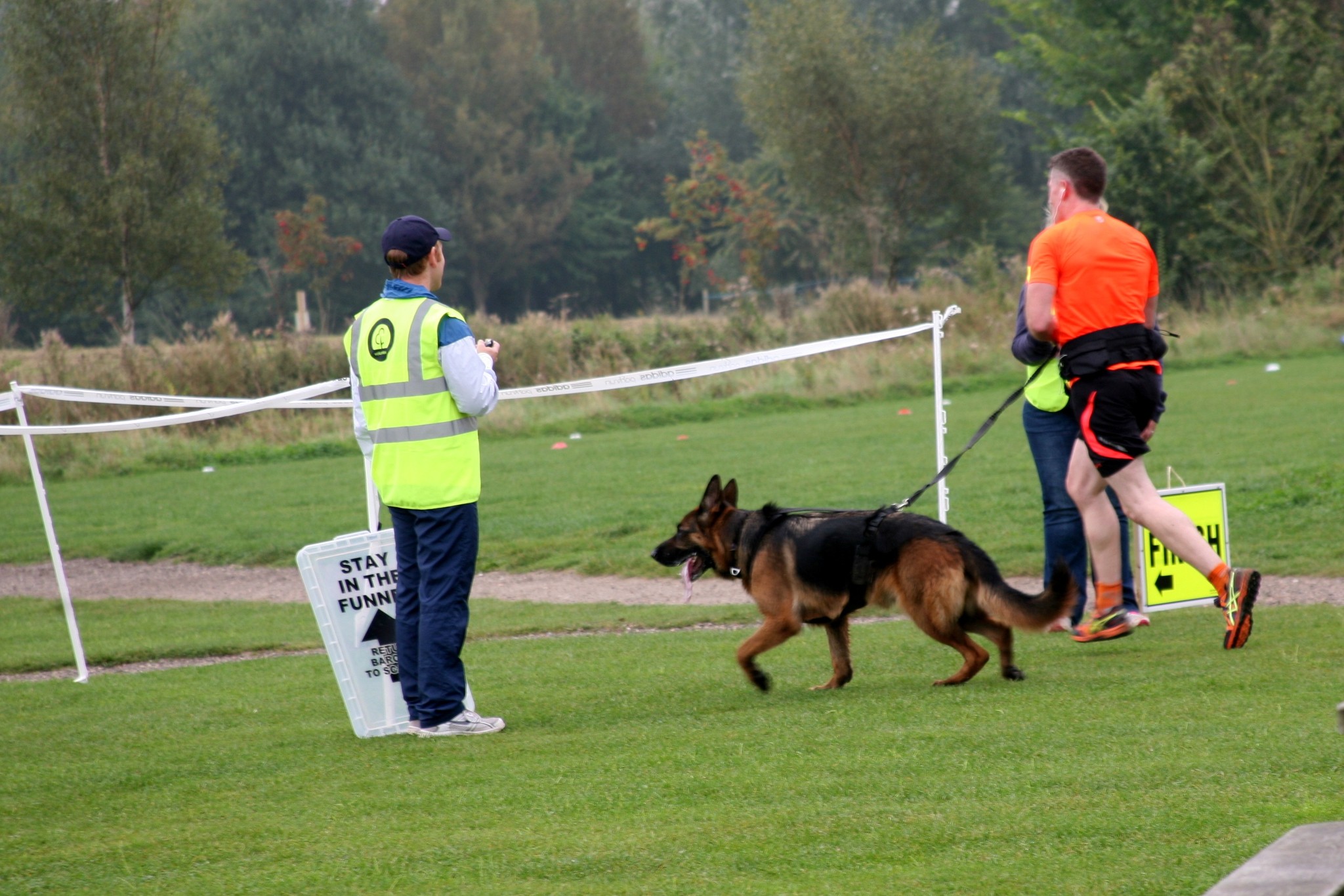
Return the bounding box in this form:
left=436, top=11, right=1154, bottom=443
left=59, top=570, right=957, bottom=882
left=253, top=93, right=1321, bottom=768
left=406, top=720, right=422, bottom=735
left=1071, top=604, right=1132, bottom=642
left=419, top=708, right=505, bottom=738
left=1214, top=568, right=1261, bottom=650
left=1127, top=610, right=1149, bottom=627
left=1046, top=616, right=1072, bottom=633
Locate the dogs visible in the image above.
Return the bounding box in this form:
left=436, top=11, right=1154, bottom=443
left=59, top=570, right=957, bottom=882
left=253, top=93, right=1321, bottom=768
left=649, top=470, right=1077, bottom=693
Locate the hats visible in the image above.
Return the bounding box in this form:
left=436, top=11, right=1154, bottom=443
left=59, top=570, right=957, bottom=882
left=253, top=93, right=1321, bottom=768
left=381, top=215, right=452, bottom=269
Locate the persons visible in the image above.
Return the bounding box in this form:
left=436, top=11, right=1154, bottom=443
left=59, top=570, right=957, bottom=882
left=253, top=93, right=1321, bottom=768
left=340, top=214, right=505, bottom=739
left=1009, top=148, right=1264, bottom=651
